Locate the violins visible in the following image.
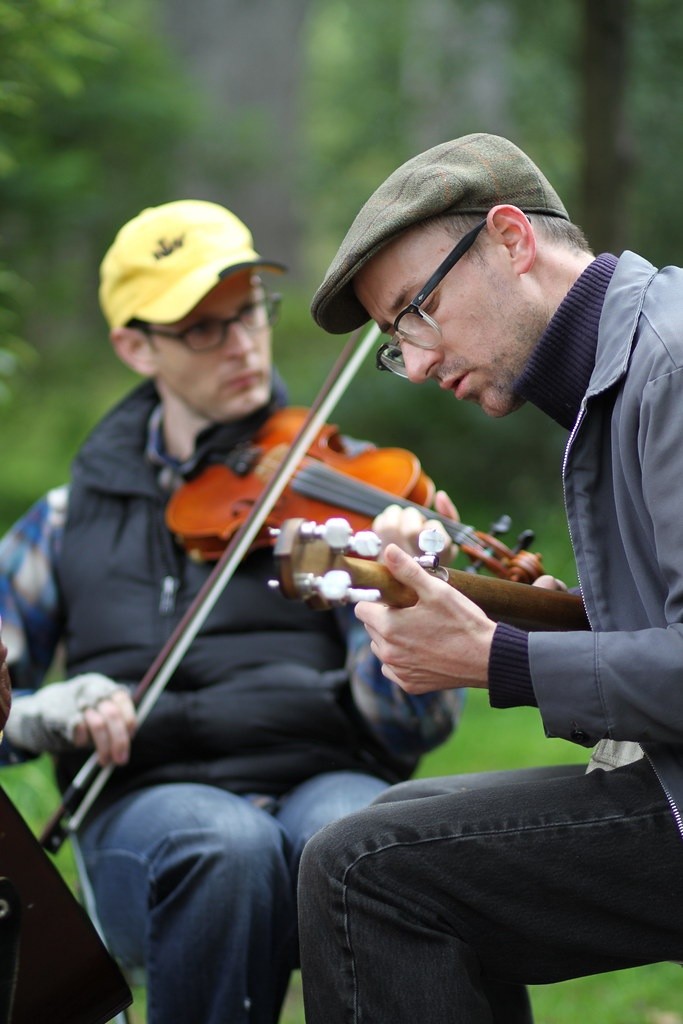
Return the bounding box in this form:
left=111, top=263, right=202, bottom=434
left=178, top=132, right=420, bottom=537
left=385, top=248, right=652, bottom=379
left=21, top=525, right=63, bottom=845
left=164, top=404, right=555, bottom=587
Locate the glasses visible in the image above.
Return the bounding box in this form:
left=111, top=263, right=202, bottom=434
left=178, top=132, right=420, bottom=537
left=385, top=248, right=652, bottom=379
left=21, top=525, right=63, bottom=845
left=375, top=215, right=531, bottom=379
left=138, top=282, right=284, bottom=353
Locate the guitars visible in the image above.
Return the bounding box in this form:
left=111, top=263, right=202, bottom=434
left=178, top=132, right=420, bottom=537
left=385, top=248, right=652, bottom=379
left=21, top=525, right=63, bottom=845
left=269, top=515, right=592, bottom=632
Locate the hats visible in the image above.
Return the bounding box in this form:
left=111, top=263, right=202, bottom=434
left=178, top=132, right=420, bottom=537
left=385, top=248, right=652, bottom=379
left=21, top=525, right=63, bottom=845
left=310, top=132, right=571, bottom=336
left=98, top=199, right=289, bottom=330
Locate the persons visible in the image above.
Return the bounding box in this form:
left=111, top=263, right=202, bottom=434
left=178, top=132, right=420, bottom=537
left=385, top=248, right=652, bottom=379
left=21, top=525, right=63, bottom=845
left=296, top=134, right=683, bottom=1024
left=0, top=198, right=455, bottom=1024
